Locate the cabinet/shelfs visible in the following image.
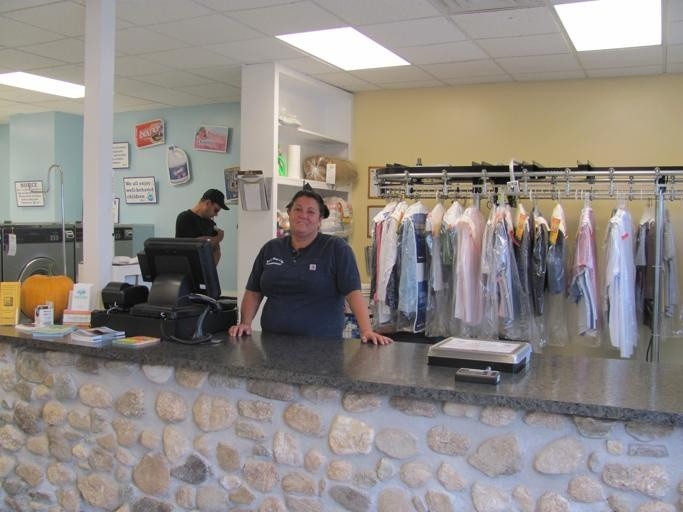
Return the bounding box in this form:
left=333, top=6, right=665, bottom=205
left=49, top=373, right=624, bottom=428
left=236, top=63, right=354, bottom=332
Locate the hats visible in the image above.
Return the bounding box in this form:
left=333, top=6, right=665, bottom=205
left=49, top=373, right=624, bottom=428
left=205, top=188, right=230, bottom=211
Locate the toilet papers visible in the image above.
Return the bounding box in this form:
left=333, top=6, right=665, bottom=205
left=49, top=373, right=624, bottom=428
left=286, top=145, right=300, bottom=177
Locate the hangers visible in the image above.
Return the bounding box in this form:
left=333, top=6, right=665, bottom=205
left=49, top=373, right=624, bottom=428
left=531, top=188, right=538, bottom=218
left=413, top=192, right=423, bottom=202
left=452, top=191, right=461, bottom=204
left=383, top=192, right=405, bottom=205
left=433, top=189, right=446, bottom=206
left=469, top=193, right=477, bottom=208
left=647, top=196, right=671, bottom=213
left=556, top=189, right=563, bottom=205
left=615, top=189, right=628, bottom=209
left=583, top=191, right=592, bottom=209
left=488, top=191, right=509, bottom=210
left=514, top=192, right=524, bottom=211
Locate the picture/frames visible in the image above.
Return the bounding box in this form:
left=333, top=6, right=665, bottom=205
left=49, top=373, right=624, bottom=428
left=222, top=165, right=241, bottom=204
left=134, top=118, right=165, bottom=151
left=123, top=175, right=157, bottom=205
left=367, top=166, right=387, bottom=200
left=14, top=179, right=45, bottom=208
left=193, top=123, right=229, bottom=155
left=366, top=204, right=386, bottom=239
left=112, top=141, right=130, bottom=169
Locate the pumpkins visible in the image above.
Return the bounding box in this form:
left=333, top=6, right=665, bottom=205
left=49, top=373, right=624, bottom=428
left=21, top=262, right=74, bottom=322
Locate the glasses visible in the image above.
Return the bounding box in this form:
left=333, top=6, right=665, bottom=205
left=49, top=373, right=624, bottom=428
left=212, top=204, right=220, bottom=214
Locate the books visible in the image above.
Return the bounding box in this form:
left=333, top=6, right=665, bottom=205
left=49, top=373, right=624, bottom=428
left=112, top=334, right=162, bottom=351
left=31, top=324, right=78, bottom=338
left=71, top=324, right=126, bottom=343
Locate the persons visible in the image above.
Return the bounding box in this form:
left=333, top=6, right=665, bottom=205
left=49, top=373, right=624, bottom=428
left=227, top=190, right=395, bottom=346
left=174, top=188, right=231, bottom=267
left=193, top=125, right=209, bottom=147
